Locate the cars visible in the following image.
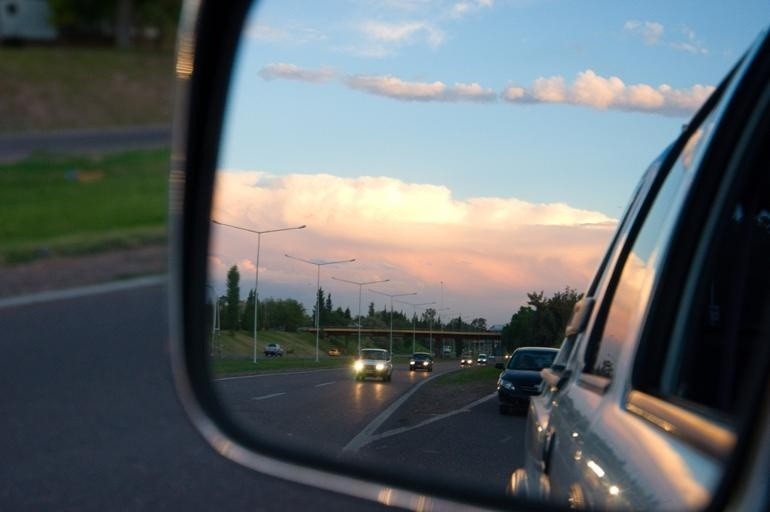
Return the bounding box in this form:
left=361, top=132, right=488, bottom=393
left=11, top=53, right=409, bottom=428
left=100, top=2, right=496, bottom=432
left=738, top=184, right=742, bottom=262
left=460, top=351, right=512, bottom=369
left=492, top=346, right=562, bottom=416
left=509, top=21, right=768, bottom=512
left=408, top=351, right=435, bottom=374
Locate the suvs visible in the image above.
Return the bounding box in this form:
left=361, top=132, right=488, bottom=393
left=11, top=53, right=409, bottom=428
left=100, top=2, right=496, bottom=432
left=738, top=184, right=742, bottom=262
left=352, top=346, right=396, bottom=384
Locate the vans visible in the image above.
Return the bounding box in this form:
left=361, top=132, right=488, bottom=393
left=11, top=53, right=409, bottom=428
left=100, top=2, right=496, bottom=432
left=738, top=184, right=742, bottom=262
left=263, top=343, right=284, bottom=358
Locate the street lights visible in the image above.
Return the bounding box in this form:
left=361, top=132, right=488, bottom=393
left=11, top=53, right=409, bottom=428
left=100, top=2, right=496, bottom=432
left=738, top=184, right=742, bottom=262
left=210, top=217, right=310, bottom=365
left=280, top=252, right=453, bottom=357
